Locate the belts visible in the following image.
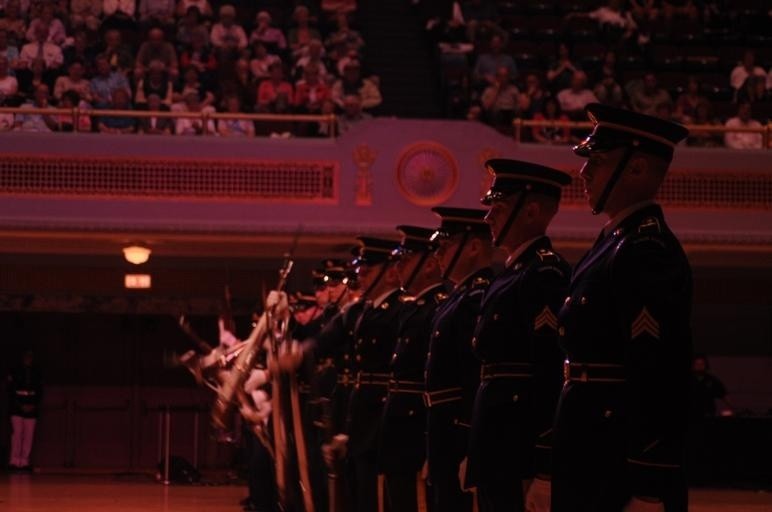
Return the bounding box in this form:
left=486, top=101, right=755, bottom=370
left=386, top=379, right=423, bottom=395
left=353, top=372, right=388, bottom=389
left=562, top=359, right=629, bottom=382
left=335, top=373, right=353, bottom=386
left=480, top=361, right=537, bottom=380
left=420, top=388, right=460, bottom=408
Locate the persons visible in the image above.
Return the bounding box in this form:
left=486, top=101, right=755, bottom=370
left=2, top=1, right=382, bottom=140
left=232, top=97, right=732, bottom=512
left=416, top=2, right=770, bottom=153
left=4, top=339, right=47, bottom=475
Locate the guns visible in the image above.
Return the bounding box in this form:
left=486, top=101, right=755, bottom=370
left=174, top=221, right=345, bottom=512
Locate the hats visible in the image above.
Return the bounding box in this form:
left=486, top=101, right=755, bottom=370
left=349, top=236, right=401, bottom=265
left=391, top=226, right=440, bottom=262
left=310, top=258, right=352, bottom=287
left=571, top=102, right=690, bottom=160
left=428, top=207, right=489, bottom=243
left=288, top=290, right=316, bottom=313
left=479, top=158, right=573, bottom=205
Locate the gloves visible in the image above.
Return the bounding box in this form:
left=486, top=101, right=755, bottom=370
left=239, top=368, right=271, bottom=432
left=199, top=348, right=226, bottom=370
left=220, top=330, right=237, bottom=348
